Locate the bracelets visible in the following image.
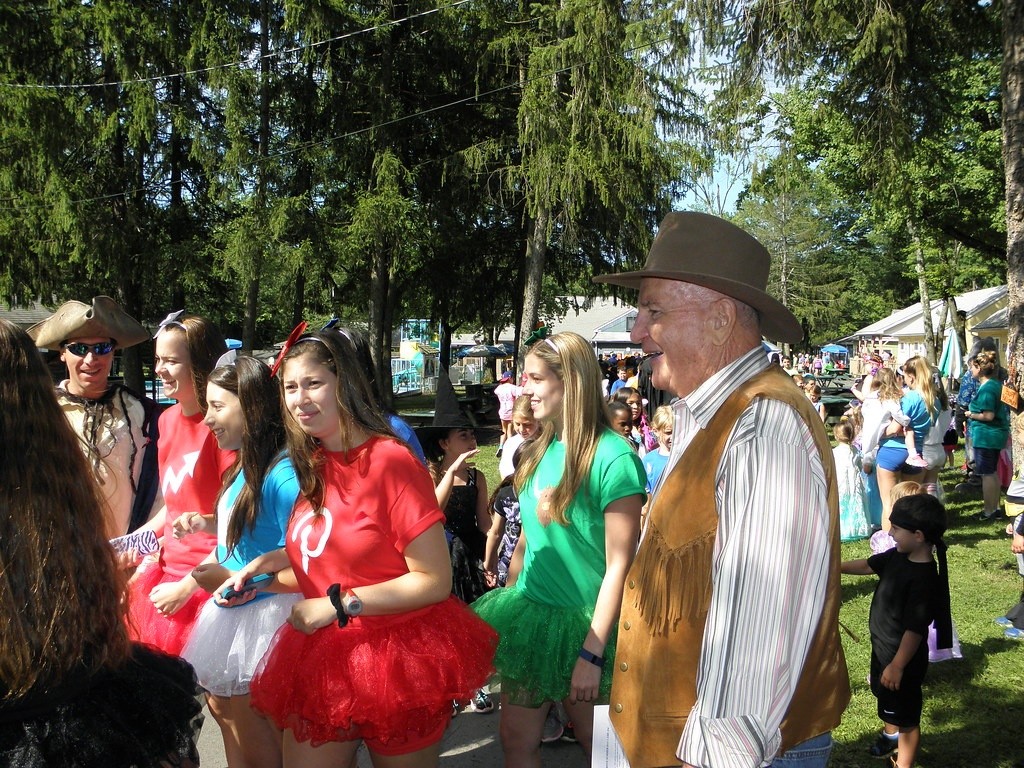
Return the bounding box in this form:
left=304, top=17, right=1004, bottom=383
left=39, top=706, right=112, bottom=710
left=969, top=414, right=972, bottom=419
left=327, top=583, right=348, bottom=627
left=579, top=647, right=606, bottom=668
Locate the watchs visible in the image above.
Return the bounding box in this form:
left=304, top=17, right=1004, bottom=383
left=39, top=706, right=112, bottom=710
left=344, top=586, right=361, bottom=618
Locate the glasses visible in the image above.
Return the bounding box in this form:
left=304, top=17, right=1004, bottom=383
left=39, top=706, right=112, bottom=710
left=64, top=338, right=114, bottom=356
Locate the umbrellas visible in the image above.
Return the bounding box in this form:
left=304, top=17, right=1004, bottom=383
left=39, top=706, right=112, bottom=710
left=495, top=343, right=514, bottom=354
left=821, top=344, right=849, bottom=356
left=455, top=345, right=506, bottom=384
left=761, top=340, right=782, bottom=353
left=938, top=332, right=961, bottom=379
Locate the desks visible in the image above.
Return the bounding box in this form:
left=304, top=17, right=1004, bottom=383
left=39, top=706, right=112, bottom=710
left=815, top=369, right=846, bottom=389
left=821, top=396, right=852, bottom=426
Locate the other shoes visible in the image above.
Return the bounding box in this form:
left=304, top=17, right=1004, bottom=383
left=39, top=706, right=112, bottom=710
left=973, top=511, right=1004, bottom=523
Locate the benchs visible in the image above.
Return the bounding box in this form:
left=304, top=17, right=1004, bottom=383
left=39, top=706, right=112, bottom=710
left=832, top=381, right=845, bottom=386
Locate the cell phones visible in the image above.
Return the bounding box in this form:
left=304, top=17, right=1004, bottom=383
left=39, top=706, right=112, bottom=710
left=221, top=572, right=275, bottom=600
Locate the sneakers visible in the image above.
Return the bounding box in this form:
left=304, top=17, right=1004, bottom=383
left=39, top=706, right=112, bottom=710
left=558, top=721, right=578, bottom=741
left=1004, top=627, right=1024, bottom=640
left=863, top=454, right=873, bottom=474
left=869, top=730, right=900, bottom=759
left=995, top=616, right=1015, bottom=628
left=471, top=690, right=494, bottom=713
left=904, top=453, right=929, bottom=467
left=540, top=701, right=563, bottom=743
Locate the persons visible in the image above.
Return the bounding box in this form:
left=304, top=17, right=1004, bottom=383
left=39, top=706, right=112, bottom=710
left=0, top=294, right=675, bottom=768
left=831, top=337, right=1023, bottom=768
left=590, top=211, right=852, bottom=768
left=782, top=352, right=833, bottom=423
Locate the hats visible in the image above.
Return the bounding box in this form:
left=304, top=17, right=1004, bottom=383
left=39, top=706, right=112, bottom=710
left=409, top=361, right=504, bottom=457
left=500, top=371, right=513, bottom=382
left=24, top=296, right=149, bottom=351
left=591, top=211, right=804, bottom=344
